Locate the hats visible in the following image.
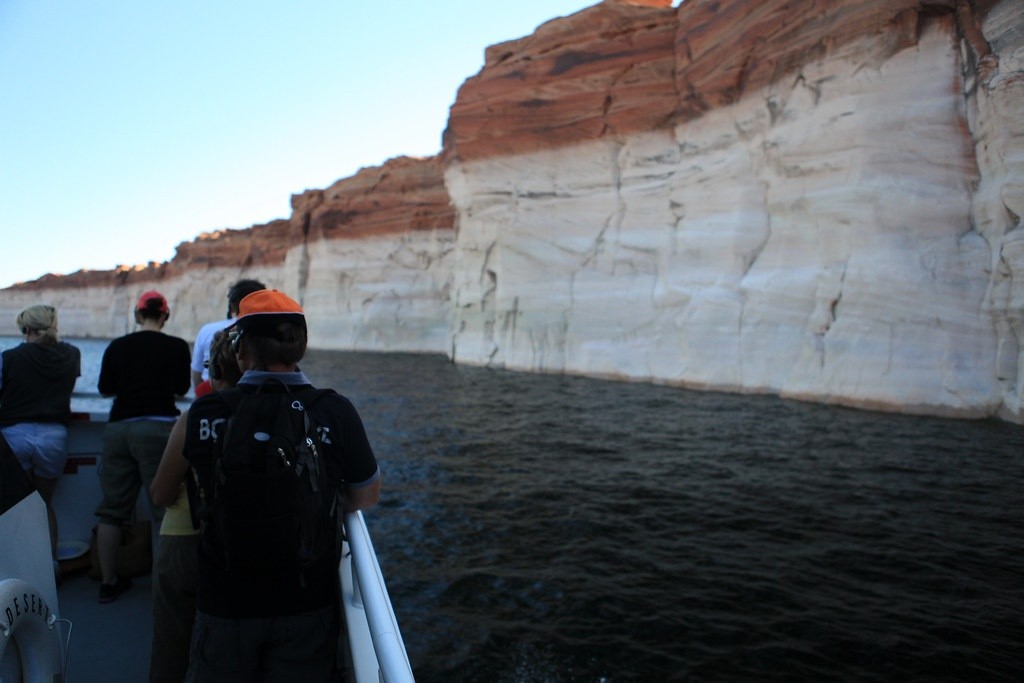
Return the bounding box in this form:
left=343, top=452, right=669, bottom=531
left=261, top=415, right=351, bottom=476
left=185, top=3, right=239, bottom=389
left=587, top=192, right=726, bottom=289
left=222, top=289, right=305, bottom=332
left=136, top=290, right=169, bottom=320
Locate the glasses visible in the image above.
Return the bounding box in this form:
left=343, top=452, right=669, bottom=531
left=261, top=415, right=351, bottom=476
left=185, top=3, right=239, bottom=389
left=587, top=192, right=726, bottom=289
left=229, top=329, right=245, bottom=354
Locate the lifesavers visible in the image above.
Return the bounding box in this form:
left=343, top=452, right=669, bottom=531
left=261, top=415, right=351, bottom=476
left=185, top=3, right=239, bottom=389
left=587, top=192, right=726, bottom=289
left=0, top=578, right=64, bottom=683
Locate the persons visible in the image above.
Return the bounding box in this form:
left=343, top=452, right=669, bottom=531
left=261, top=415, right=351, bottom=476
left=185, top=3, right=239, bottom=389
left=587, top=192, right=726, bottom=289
left=151, top=328, right=241, bottom=683
left=182, top=290, right=381, bottom=682
left=94, top=290, right=192, bottom=603
left=0, top=305, right=81, bottom=579
left=190, top=280, right=267, bottom=394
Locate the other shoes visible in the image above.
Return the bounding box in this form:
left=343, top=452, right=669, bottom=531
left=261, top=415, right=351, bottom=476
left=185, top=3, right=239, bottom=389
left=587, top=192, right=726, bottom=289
left=52, top=560, right=71, bottom=583
left=99, top=573, right=123, bottom=603
